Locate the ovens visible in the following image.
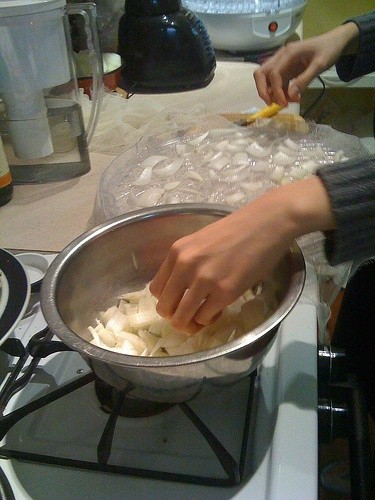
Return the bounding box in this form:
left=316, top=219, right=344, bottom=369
left=318, top=347, right=374, bottom=500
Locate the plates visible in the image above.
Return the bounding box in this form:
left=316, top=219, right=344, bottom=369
left=96, top=117, right=370, bottom=253
left=0, top=248, right=31, bottom=346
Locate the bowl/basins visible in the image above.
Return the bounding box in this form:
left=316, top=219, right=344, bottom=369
left=39, top=202, right=307, bottom=404
left=14, top=253, right=50, bottom=289
left=51, top=52, right=126, bottom=97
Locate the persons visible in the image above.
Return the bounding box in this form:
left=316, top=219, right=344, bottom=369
left=150, top=11, right=375, bottom=334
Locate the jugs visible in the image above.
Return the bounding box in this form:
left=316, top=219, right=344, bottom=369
left=0, top=0, right=104, bottom=183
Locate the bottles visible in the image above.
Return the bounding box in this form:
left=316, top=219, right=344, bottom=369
left=118, top=0, right=217, bottom=87
left=0, top=136, right=14, bottom=206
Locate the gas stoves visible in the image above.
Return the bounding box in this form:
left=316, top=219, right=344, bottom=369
left=0, top=247, right=354, bottom=500
